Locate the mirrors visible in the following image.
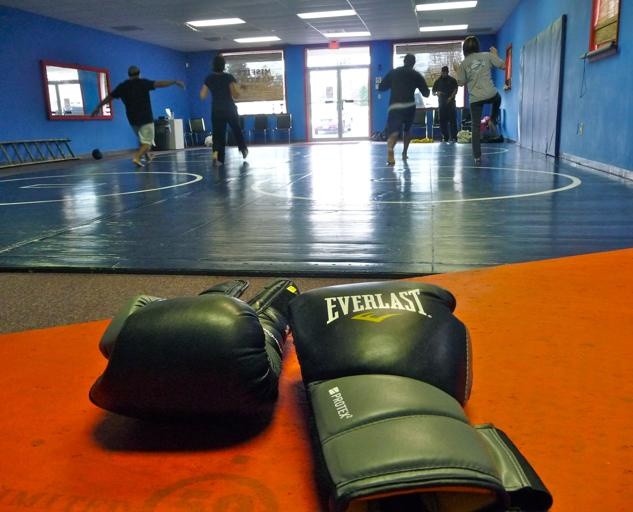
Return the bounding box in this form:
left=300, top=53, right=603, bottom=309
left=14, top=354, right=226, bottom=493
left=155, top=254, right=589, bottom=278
left=39, top=59, right=114, bottom=121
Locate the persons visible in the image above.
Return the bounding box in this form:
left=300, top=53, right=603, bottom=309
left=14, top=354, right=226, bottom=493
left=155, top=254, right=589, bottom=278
left=90, top=65, right=186, bottom=167
left=378, top=52, right=431, bottom=164
left=199, top=55, right=249, bottom=166
left=431, top=66, right=459, bottom=142
left=453, top=35, right=507, bottom=166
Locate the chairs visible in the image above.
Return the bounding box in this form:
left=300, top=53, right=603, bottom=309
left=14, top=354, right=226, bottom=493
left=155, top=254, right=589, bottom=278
left=404, top=108, right=463, bottom=142
left=237, top=113, right=293, bottom=145
left=188, top=118, right=212, bottom=146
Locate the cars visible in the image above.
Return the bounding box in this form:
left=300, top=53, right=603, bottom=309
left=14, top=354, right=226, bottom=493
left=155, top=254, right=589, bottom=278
left=314, top=116, right=350, bottom=135
